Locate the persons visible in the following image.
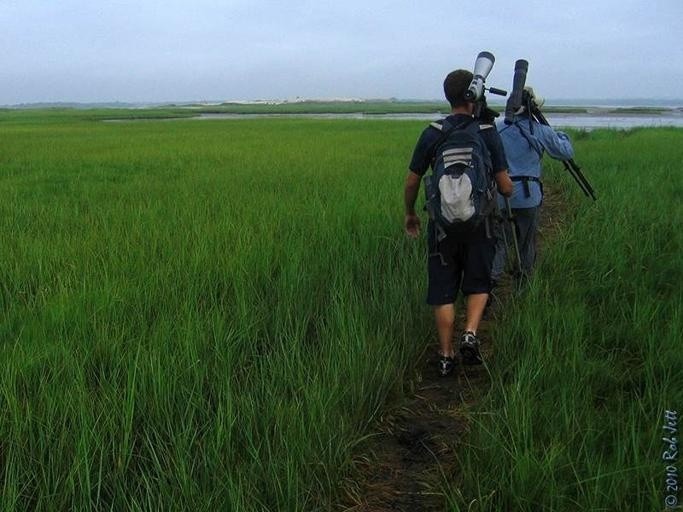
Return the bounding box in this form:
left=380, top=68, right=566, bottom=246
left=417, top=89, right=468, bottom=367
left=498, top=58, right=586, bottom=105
left=481, top=84, right=575, bottom=323
left=403, top=69, right=514, bottom=376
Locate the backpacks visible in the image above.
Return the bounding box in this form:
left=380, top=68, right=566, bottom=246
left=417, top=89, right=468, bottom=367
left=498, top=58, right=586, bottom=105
left=423, top=119, right=498, bottom=238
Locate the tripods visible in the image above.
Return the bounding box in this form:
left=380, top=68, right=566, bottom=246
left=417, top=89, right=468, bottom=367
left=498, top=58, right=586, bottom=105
left=525, top=93, right=598, bottom=202
left=476, top=104, right=525, bottom=277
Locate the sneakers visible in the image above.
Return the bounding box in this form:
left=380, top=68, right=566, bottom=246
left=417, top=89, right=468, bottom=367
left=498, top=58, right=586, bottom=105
left=460, top=332, right=483, bottom=365
left=437, top=350, right=457, bottom=378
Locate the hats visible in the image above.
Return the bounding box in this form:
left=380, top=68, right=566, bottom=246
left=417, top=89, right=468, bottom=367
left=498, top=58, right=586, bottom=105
left=523, top=86, right=545, bottom=110
